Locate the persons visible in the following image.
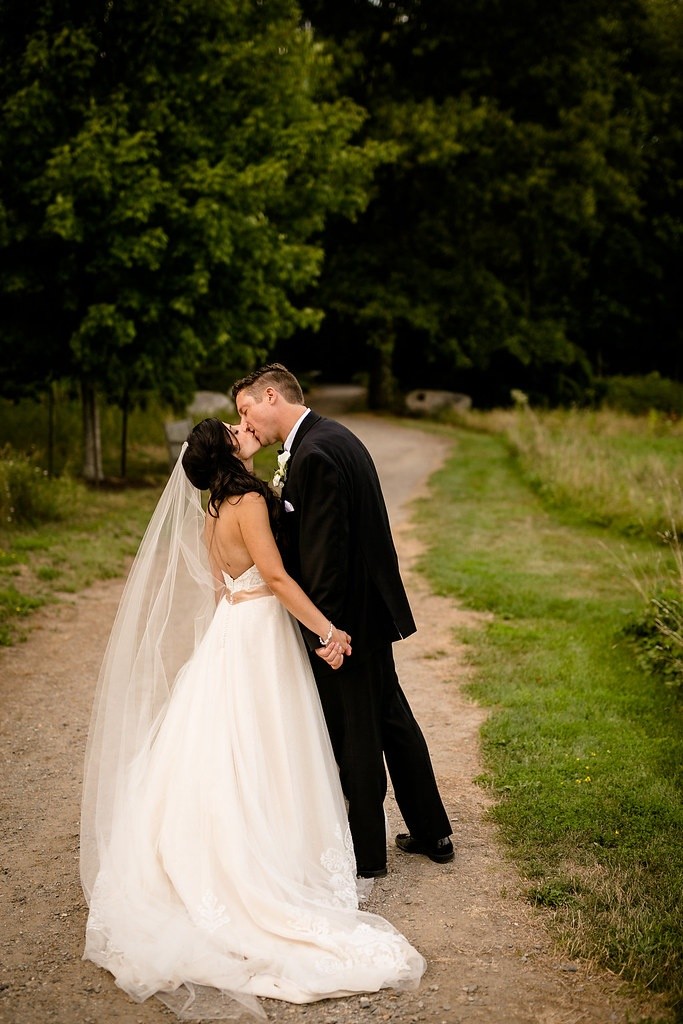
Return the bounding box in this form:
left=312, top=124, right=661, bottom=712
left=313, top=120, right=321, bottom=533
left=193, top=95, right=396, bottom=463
left=232, top=362, right=455, bottom=879
left=78, top=417, right=427, bottom=1023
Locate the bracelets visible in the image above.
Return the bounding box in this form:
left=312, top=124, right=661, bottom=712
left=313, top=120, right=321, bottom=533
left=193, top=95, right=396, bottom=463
left=319, top=621, right=333, bottom=645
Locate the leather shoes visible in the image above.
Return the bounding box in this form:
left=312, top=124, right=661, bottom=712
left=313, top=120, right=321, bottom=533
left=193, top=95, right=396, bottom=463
left=395, top=833, right=455, bottom=864
left=355, top=861, right=387, bottom=879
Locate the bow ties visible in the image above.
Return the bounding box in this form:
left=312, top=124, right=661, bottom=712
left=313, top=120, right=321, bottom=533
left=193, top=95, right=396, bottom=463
left=277, top=444, right=284, bottom=455
